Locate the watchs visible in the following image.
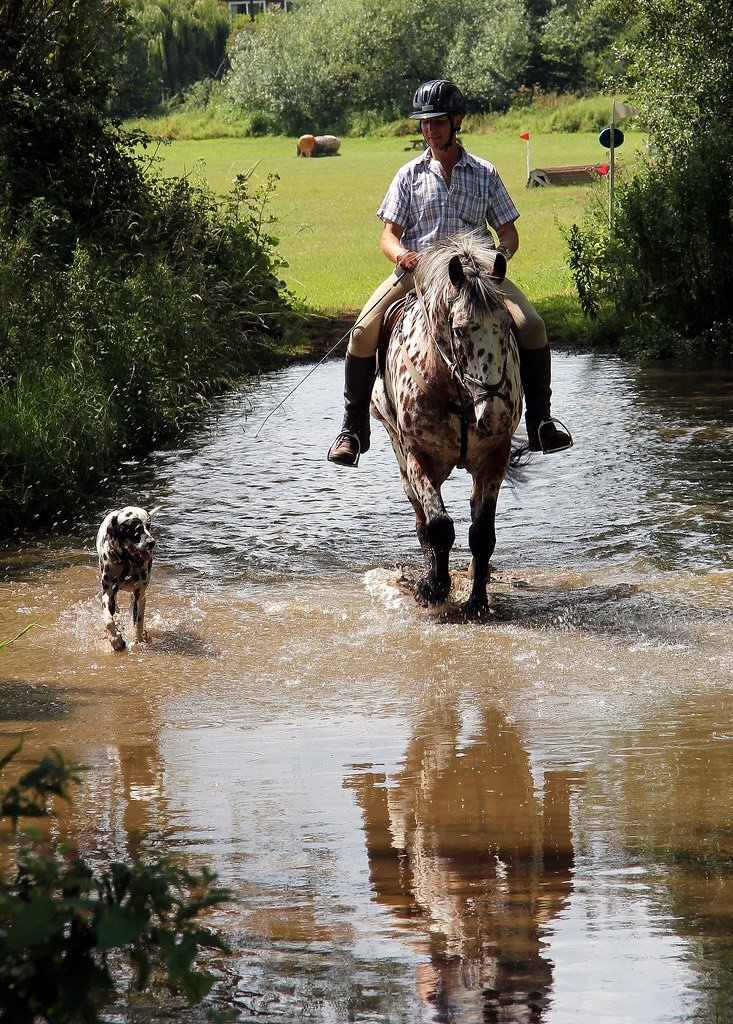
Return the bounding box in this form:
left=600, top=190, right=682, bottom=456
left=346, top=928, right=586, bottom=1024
left=497, top=246, right=512, bottom=259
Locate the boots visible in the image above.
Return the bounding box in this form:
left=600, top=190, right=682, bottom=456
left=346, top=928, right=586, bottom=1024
left=328, top=349, right=376, bottom=462
left=515, top=342, right=570, bottom=452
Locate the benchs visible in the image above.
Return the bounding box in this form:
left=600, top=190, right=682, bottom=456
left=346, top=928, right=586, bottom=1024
left=410, top=137, right=463, bottom=151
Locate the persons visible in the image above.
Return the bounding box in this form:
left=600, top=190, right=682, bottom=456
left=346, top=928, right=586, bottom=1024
left=329, top=79, right=573, bottom=469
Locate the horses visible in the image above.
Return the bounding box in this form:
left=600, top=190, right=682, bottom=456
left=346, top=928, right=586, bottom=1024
left=369, top=224, right=534, bottom=625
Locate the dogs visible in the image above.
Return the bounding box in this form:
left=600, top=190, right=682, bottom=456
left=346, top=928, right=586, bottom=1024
left=95, top=504, right=165, bottom=654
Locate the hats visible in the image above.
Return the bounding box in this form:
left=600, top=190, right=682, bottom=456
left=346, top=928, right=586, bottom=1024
left=408, top=80, right=465, bottom=123
